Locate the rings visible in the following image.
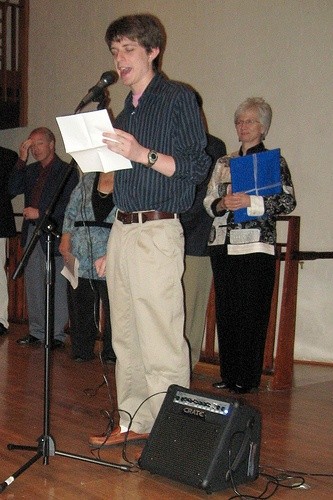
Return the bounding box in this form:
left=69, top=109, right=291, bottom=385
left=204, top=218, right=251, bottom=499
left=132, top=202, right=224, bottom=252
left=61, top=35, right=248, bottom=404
left=25, top=214, right=26, bottom=217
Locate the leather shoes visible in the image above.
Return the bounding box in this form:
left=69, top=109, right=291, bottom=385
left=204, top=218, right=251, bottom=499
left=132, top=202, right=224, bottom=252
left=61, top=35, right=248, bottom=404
left=213, top=381, right=230, bottom=388
left=16, top=334, right=39, bottom=343
left=229, top=383, right=260, bottom=394
left=89, top=425, right=153, bottom=445
left=103, top=346, right=117, bottom=364
left=51, top=339, right=65, bottom=350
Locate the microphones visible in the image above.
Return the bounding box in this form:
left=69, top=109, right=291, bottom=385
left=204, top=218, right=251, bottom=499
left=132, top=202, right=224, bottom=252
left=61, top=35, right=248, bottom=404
left=75, top=72, right=116, bottom=112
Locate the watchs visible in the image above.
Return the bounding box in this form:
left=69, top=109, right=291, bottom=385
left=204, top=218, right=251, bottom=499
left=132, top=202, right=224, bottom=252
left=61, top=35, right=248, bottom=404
left=146, top=150, right=157, bottom=167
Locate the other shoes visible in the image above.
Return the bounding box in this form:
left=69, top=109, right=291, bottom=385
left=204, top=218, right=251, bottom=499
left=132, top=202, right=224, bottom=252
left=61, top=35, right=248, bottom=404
left=82, top=353, right=94, bottom=360
left=69, top=348, right=81, bottom=360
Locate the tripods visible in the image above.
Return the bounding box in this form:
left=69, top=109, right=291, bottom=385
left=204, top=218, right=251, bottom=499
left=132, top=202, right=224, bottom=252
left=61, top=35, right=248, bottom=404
left=0, top=92, right=130, bottom=493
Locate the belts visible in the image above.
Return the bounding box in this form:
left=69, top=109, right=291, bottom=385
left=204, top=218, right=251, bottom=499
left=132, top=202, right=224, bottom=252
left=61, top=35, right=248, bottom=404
left=115, top=207, right=176, bottom=225
left=74, top=221, right=113, bottom=228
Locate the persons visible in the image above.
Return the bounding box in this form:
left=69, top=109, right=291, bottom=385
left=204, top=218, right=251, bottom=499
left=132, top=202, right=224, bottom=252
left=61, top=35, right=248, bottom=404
left=204, top=98, right=293, bottom=395
left=7, top=127, right=80, bottom=349
left=58, top=170, right=117, bottom=364
left=0, top=146, right=19, bottom=334
left=89, top=14, right=210, bottom=445
left=177, top=133, right=227, bottom=370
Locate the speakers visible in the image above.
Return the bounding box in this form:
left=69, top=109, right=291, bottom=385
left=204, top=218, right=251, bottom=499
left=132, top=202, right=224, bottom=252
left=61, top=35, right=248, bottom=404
left=139, top=383, right=262, bottom=495
